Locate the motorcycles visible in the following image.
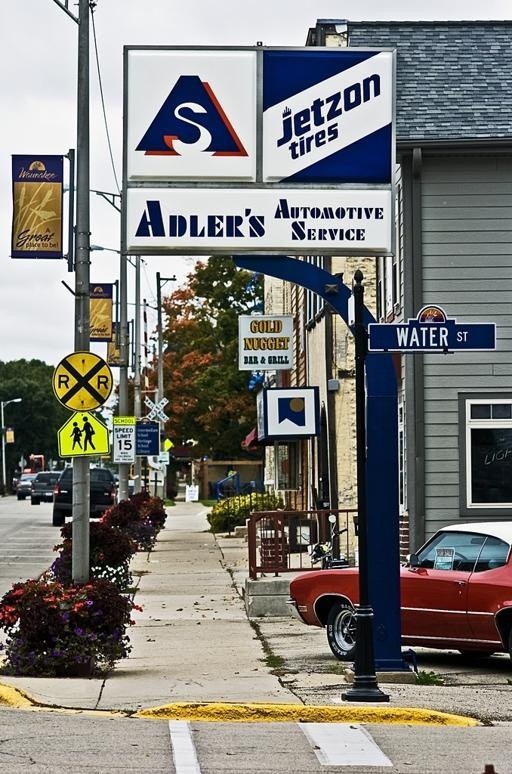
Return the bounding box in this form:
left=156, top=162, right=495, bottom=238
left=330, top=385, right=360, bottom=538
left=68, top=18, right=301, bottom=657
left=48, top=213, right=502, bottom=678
left=301, top=513, right=350, bottom=569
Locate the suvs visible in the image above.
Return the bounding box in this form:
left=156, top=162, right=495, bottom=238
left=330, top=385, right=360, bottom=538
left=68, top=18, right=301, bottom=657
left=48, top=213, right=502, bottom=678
left=52, top=465, right=118, bottom=526
left=31, top=470, right=64, bottom=504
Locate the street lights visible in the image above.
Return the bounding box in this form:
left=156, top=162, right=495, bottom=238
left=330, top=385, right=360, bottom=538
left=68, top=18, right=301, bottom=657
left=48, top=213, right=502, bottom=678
left=62, top=183, right=129, bottom=509
left=84, top=243, right=145, bottom=495
left=1, top=397, right=23, bottom=497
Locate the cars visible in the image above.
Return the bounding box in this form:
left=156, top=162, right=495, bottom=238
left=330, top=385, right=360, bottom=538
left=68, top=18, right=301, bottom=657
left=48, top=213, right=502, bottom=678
left=285, top=519, right=512, bottom=666
left=13, top=472, right=39, bottom=500
